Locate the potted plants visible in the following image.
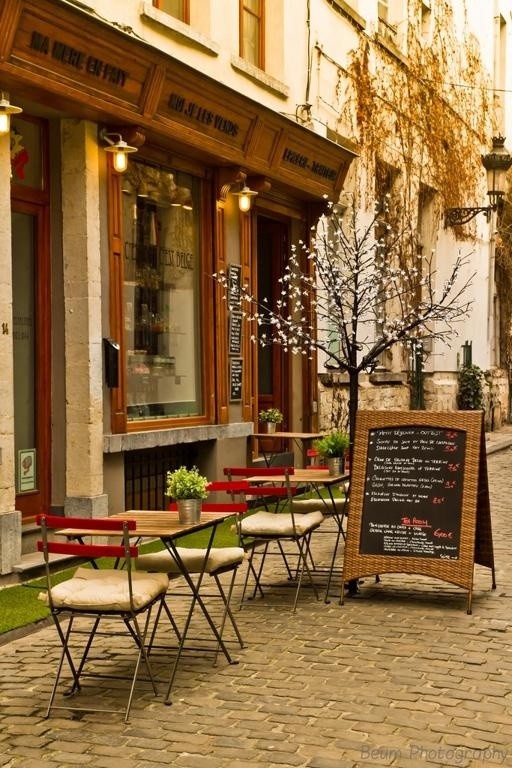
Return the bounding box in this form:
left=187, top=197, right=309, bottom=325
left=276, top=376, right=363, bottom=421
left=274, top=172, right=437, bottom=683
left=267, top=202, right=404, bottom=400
left=258, top=408, right=283, bottom=434
left=311, top=431, right=349, bottom=476
left=163, top=464, right=212, bottom=525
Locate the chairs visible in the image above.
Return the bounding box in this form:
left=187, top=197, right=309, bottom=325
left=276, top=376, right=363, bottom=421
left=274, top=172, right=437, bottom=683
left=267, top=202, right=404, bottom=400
left=36, top=513, right=169, bottom=722
left=293, top=448, right=380, bottom=583
left=222, top=466, right=326, bottom=614
left=137, top=480, right=249, bottom=668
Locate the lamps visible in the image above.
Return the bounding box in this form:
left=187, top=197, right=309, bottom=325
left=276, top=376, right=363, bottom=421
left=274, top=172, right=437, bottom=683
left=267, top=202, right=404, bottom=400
left=444, top=132, right=512, bottom=230
left=218, top=171, right=259, bottom=212
left=99, top=128, right=138, bottom=173
left=0, top=91, right=23, bottom=133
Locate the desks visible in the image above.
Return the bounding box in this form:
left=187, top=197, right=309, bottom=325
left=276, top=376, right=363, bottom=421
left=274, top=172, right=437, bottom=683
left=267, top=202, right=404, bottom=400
left=242, top=468, right=351, bottom=604
left=54, top=509, right=238, bottom=705
left=252, top=431, right=329, bottom=467
